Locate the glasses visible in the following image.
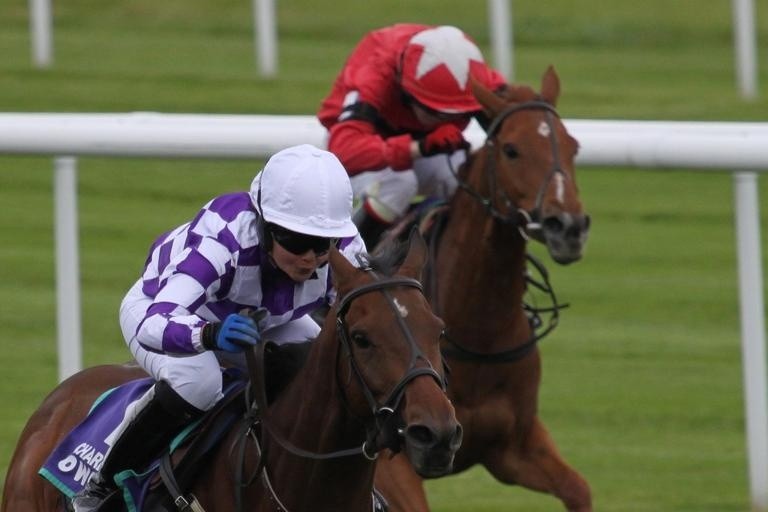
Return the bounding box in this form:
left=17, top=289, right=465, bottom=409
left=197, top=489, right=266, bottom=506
left=270, top=226, right=332, bottom=257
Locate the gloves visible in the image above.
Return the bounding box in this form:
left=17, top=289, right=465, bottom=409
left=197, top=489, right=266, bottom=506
left=420, top=124, right=468, bottom=158
left=199, top=312, right=260, bottom=356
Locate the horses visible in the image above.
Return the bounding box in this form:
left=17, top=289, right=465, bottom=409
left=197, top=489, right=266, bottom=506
left=373, top=64, right=592, bottom=512
left=0, top=225, right=463, bottom=512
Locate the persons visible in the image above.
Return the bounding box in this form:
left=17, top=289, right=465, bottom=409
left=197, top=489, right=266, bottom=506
left=314, top=23, right=508, bottom=255
left=70, top=140, right=372, bottom=511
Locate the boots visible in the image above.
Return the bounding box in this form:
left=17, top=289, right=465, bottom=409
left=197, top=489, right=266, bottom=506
left=355, top=200, right=388, bottom=256
left=71, top=382, right=206, bottom=512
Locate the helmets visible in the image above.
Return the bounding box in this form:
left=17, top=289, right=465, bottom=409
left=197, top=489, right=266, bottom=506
left=249, top=146, right=359, bottom=240
left=399, top=24, right=493, bottom=116
